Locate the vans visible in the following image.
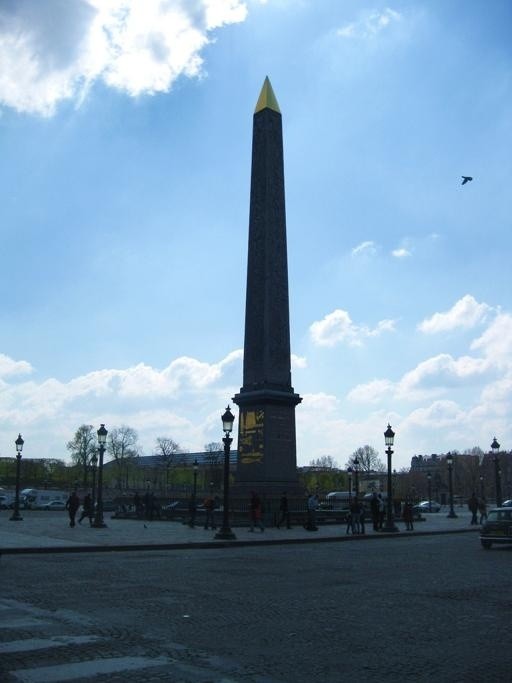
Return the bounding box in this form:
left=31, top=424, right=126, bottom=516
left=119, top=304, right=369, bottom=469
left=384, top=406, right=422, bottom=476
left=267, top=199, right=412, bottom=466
left=321, top=492, right=357, bottom=509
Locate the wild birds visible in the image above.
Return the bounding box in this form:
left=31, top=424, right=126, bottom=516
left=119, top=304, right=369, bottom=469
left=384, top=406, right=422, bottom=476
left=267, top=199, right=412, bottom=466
left=461, top=176, right=472, bottom=185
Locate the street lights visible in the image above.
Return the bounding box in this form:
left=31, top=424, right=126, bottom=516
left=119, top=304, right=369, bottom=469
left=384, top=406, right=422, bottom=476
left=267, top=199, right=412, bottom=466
left=381, top=422, right=399, bottom=532
left=214, top=404, right=235, bottom=539
left=88, top=425, right=108, bottom=528
left=10, top=434, right=24, bottom=520
left=348, top=456, right=359, bottom=506
left=188, top=459, right=200, bottom=528
left=428, top=436, right=502, bottom=520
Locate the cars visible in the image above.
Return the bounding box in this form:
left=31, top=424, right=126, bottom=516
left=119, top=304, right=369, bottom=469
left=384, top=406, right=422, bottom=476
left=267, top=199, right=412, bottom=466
left=41, top=501, right=70, bottom=511
left=415, top=500, right=441, bottom=513
left=480, top=499, right=512, bottom=547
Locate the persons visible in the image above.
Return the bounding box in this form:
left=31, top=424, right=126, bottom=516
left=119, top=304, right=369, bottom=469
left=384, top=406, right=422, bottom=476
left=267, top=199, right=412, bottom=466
left=133, top=490, right=161, bottom=521
left=202, top=495, right=218, bottom=531
left=78, top=492, right=96, bottom=526
left=275, top=491, right=293, bottom=530
left=245, top=488, right=265, bottom=532
left=302, top=492, right=321, bottom=531
left=187, top=492, right=197, bottom=527
left=65, top=490, right=80, bottom=528
left=467, top=492, right=479, bottom=525
left=478, top=495, right=490, bottom=525
left=341, top=491, right=414, bottom=534
